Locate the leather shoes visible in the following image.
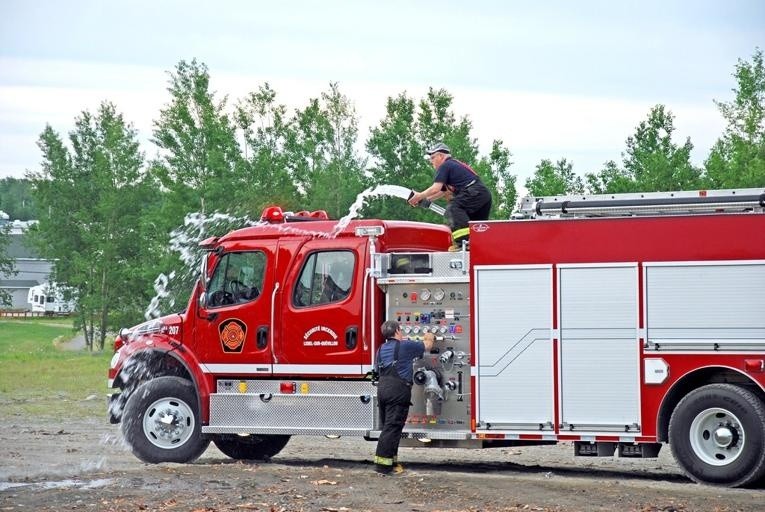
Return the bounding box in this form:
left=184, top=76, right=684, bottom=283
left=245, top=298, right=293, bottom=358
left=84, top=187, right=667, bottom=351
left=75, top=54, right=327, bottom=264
left=377, top=467, right=404, bottom=474
left=448, top=243, right=462, bottom=251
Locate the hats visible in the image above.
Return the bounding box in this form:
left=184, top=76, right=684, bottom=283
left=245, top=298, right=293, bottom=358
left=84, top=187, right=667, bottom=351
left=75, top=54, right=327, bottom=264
left=425, top=143, right=451, bottom=155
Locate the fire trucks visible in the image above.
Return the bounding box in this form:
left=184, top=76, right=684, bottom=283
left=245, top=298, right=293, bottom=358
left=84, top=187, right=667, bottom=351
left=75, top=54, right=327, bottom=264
left=108, top=187, right=765, bottom=487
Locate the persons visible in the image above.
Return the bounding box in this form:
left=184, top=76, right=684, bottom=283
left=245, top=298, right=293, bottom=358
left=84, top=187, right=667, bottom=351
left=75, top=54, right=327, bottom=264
left=373, top=320, right=435, bottom=474
left=408, top=143, right=492, bottom=251
